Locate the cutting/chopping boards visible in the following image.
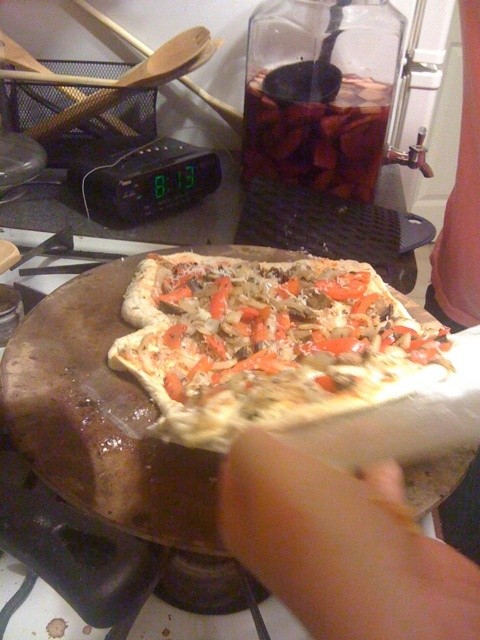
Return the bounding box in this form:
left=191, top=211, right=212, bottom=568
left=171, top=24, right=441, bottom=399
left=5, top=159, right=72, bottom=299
left=0, top=244, right=477, bottom=558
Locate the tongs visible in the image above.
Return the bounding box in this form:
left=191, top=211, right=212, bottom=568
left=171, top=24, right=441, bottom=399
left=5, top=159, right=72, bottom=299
left=17, top=246, right=129, bottom=276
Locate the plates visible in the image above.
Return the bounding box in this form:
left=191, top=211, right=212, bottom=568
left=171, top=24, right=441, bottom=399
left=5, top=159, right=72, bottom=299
left=276, top=324, right=480, bottom=477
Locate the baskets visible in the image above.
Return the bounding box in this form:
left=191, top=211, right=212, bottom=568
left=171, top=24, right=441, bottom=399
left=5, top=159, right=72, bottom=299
left=0, top=60, right=158, bottom=168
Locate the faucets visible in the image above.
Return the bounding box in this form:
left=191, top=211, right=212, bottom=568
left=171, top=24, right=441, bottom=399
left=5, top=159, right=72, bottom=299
left=381, top=126, right=434, bottom=178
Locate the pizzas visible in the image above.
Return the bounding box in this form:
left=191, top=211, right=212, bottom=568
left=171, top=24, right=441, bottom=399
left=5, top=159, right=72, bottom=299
left=107, top=254, right=449, bottom=453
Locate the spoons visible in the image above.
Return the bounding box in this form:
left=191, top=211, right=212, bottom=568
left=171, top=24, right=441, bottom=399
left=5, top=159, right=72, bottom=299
left=0, top=24, right=226, bottom=143
left=261, top=0, right=352, bottom=103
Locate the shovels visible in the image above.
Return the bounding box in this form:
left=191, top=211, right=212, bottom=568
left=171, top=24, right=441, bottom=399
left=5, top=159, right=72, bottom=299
left=75, top=0, right=245, bottom=138
left=31, top=40, right=225, bottom=138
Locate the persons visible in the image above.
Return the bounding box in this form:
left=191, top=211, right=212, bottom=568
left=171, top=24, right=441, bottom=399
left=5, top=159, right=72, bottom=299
left=218, top=426, right=479, bottom=638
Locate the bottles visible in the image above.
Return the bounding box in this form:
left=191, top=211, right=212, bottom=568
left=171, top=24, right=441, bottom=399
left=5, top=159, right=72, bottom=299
left=243, top=2, right=408, bottom=202
left=0, top=283, right=25, bottom=347
